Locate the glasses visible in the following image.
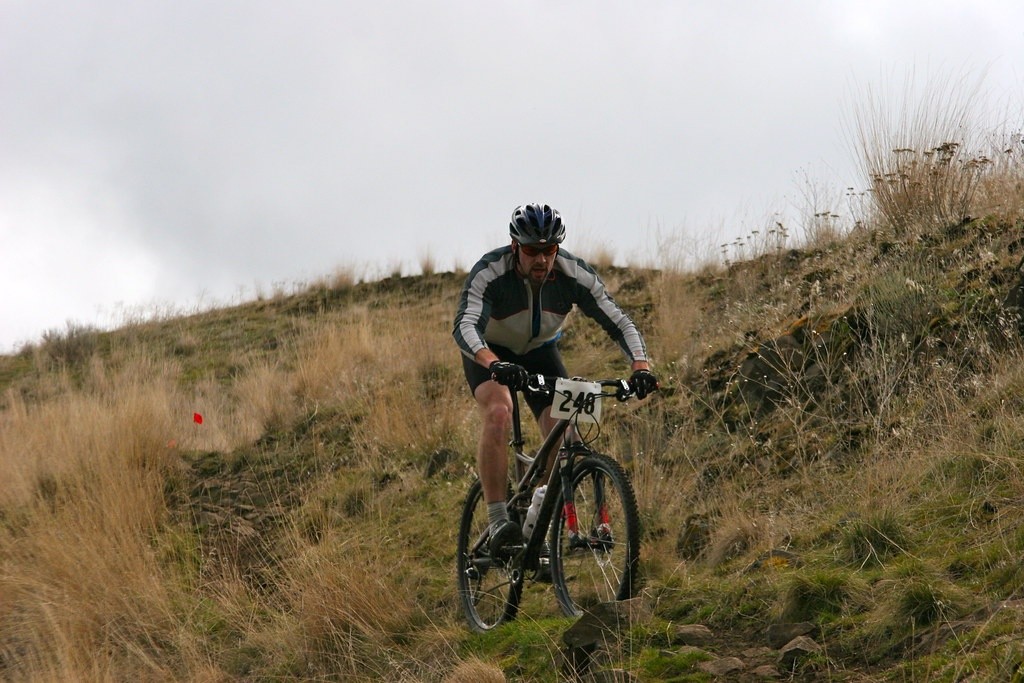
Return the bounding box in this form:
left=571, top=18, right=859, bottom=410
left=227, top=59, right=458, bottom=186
left=518, top=243, right=558, bottom=257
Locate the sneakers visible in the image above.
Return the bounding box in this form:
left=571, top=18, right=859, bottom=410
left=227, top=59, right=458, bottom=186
left=537, top=542, right=552, bottom=568
left=488, top=519, right=522, bottom=567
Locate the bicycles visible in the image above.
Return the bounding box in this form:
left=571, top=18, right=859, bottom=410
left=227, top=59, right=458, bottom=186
left=456, top=371, right=660, bottom=635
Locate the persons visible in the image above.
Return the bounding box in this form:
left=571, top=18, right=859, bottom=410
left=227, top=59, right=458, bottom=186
left=452, top=203, right=662, bottom=584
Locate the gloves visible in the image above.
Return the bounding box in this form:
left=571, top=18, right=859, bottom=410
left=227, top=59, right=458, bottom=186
left=489, top=361, right=529, bottom=391
left=627, top=369, right=658, bottom=399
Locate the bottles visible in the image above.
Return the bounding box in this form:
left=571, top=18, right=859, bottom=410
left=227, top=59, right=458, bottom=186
left=522, top=485, right=548, bottom=539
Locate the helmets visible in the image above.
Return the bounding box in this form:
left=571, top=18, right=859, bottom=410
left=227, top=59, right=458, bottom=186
left=508, top=202, right=565, bottom=243
